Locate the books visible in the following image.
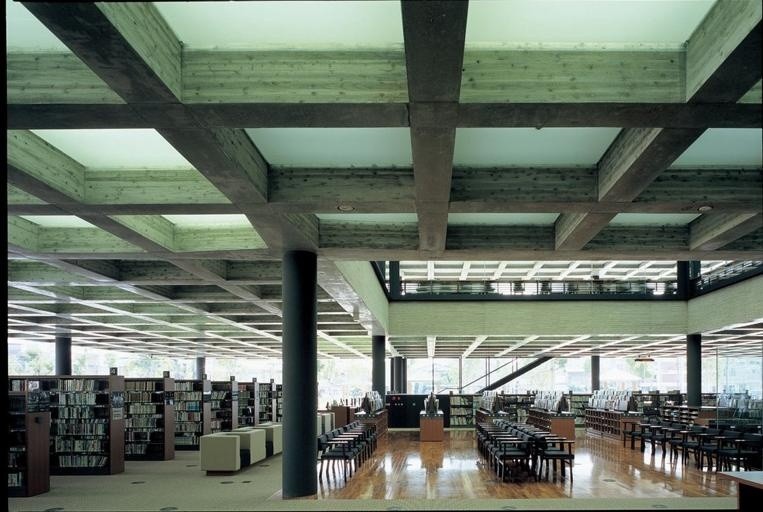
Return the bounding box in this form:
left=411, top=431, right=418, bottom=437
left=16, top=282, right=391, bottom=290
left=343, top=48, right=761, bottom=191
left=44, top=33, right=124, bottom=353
left=449, top=390, right=698, bottom=430
left=172, top=381, right=283, bottom=446
left=4, top=377, right=164, bottom=489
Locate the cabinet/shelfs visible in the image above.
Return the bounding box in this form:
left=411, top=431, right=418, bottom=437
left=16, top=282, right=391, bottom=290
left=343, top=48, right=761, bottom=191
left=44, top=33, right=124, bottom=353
left=9, top=375, right=278, bottom=497
left=450, top=392, right=738, bottom=441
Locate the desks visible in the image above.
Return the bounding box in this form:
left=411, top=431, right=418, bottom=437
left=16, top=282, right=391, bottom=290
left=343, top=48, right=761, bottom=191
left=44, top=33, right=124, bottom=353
left=717, top=471, right=763, bottom=512
left=420, top=408, right=444, bottom=441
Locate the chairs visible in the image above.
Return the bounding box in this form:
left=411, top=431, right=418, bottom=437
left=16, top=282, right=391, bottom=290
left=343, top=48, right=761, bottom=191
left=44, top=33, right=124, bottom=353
left=536, top=435, right=575, bottom=483
left=495, top=441, right=539, bottom=483
left=718, top=433, right=763, bottom=472
left=318, top=420, right=378, bottom=482
